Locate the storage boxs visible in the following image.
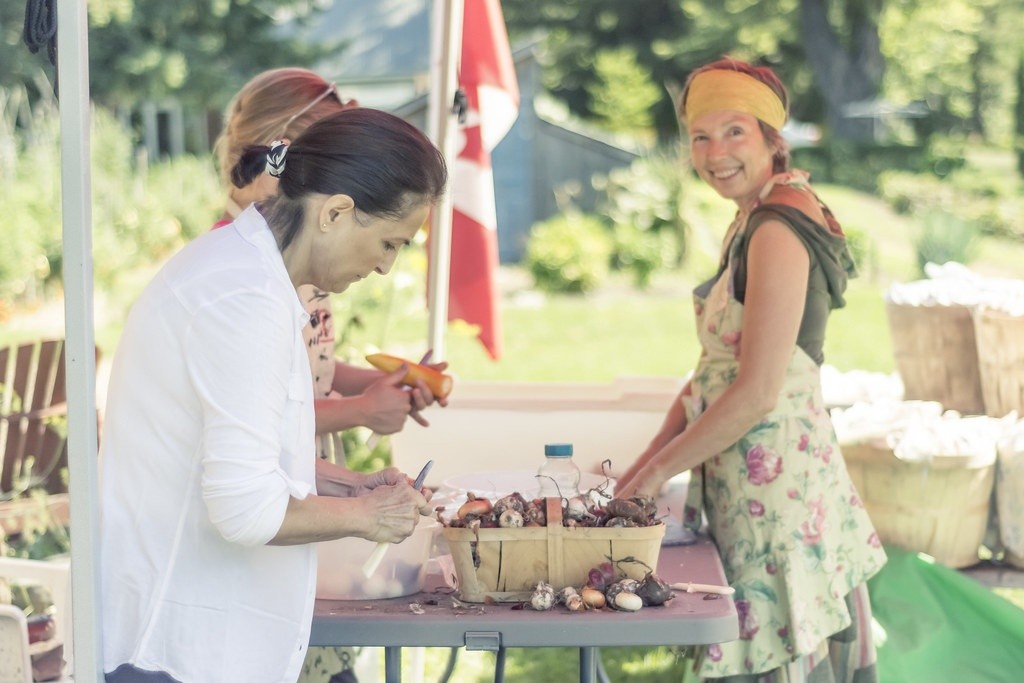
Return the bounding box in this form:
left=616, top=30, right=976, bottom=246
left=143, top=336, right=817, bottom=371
left=315, top=513, right=446, bottom=600
left=830, top=279, right=1024, bottom=568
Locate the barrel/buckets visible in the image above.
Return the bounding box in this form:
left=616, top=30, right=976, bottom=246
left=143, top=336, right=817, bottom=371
left=840, top=298, right=1024, bottom=568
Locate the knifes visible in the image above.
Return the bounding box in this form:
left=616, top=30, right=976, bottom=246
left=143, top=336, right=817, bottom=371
left=363, top=458, right=434, bottom=579
left=365, top=350, right=433, bottom=450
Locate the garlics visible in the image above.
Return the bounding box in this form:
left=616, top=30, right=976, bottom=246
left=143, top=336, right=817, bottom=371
left=444, top=492, right=672, bottom=611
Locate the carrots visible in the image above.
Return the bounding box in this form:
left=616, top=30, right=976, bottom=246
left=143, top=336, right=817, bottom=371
left=365, top=353, right=453, bottom=398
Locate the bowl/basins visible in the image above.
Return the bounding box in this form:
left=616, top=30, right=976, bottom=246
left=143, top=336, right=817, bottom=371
left=316, top=516, right=440, bottom=599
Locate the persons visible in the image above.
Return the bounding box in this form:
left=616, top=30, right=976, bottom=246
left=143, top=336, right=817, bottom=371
left=612, top=60, right=887, bottom=683
left=100, top=108, right=448, bottom=683
left=213, top=67, right=449, bottom=683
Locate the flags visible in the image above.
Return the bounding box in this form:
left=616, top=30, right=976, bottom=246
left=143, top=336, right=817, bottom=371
left=422, top=0, right=519, bottom=362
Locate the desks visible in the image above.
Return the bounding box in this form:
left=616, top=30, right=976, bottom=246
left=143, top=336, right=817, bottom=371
left=307, top=521, right=739, bottom=683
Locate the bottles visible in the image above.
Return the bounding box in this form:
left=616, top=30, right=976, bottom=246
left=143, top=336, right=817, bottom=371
left=537, top=443, right=581, bottom=500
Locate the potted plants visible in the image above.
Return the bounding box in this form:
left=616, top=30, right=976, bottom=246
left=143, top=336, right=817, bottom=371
left=10, top=579, right=55, bottom=644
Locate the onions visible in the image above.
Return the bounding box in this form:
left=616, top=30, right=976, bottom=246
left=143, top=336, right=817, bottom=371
left=457, top=492, right=492, bottom=520
